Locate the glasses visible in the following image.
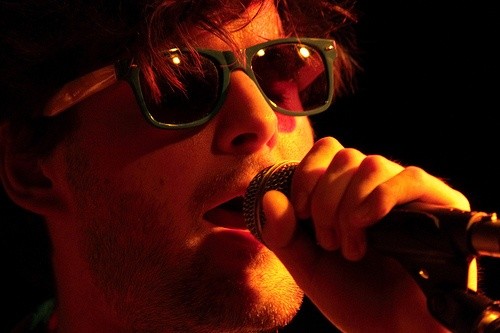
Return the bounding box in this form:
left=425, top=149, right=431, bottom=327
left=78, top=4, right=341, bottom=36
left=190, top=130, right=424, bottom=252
left=41, top=38, right=338, bottom=130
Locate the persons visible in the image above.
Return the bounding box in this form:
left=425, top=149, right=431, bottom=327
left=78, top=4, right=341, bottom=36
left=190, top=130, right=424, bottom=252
left=0, top=1, right=478, bottom=333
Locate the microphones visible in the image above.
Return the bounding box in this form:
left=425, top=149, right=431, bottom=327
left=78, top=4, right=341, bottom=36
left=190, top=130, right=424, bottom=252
left=243, top=160, right=500, bottom=264
